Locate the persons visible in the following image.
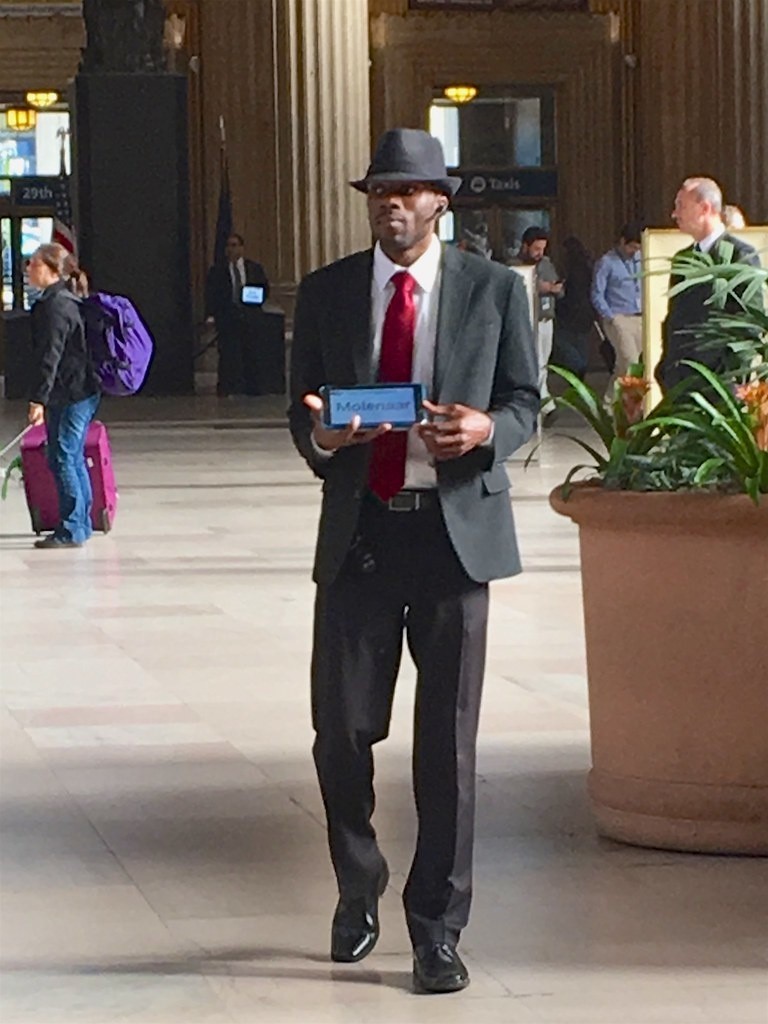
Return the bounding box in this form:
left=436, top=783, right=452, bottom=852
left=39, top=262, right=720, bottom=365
left=458, top=226, right=641, bottom=430
left=654, top=178, right=765, bottom=406
left=27, top=243, right=101, bottom=548
left=206, top=233, right=270, bottom=397
left=720, top=205, right=744, bottom=228
left=289, top=128, right=540, bottom=990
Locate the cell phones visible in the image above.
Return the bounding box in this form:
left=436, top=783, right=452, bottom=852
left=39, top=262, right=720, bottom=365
left=554, top=280, right=564, bottom=285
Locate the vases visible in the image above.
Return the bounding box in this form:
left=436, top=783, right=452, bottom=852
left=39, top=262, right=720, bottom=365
left=550, top=477, right=767, bottom=853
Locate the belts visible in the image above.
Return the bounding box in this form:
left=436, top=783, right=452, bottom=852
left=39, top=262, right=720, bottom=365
left=365, top=490, right=439, bottom=513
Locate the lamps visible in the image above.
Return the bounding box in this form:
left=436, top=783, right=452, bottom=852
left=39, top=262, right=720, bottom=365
left=5, top=91, right=58, bottom=131
left=444, top=84, right=478, bottom=103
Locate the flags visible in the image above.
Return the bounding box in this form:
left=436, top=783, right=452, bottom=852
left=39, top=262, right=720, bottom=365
left=51, top=170, right=75, bottom=258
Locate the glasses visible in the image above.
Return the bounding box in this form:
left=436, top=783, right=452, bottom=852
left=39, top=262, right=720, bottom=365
left=225, top=243, right=237, bottom=248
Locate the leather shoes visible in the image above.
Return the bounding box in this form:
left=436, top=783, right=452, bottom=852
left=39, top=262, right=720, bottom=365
left=331, top=860, right=388, bottom=962
left=413, top=942, right=470, bottom=993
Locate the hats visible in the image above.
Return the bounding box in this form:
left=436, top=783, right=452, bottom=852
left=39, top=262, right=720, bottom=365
left=350, top=129, right=463, bottom=200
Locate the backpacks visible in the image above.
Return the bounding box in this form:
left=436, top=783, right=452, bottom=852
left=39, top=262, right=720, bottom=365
left=56, top=289, right=152, bottom=395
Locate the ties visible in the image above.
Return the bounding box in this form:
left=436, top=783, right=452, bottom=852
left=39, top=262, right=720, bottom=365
left=368, top=272, right=415, bottom=501
left=233, top=263, right=241, bottom=302
left=693, top=244, right=702, bottom=261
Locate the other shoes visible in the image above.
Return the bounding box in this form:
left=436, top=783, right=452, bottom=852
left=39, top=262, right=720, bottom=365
left=35, top=535, right=81, bottom=548
left=217, top=383, right=230, bottom=399
left=542, top=410, right=557, bottom=428
left=239, top=384, right=259, bottom=397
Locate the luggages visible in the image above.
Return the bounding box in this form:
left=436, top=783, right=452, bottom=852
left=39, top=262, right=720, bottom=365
left=19, top=417, right=117, bottom=533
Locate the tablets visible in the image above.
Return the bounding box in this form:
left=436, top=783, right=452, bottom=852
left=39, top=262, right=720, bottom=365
left=242, top=285, right=266, bottom=305
left=318, top=382, right=428, bottom=427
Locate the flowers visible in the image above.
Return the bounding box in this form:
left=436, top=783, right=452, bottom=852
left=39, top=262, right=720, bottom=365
left=518, top=241, right=768, bottom=501
